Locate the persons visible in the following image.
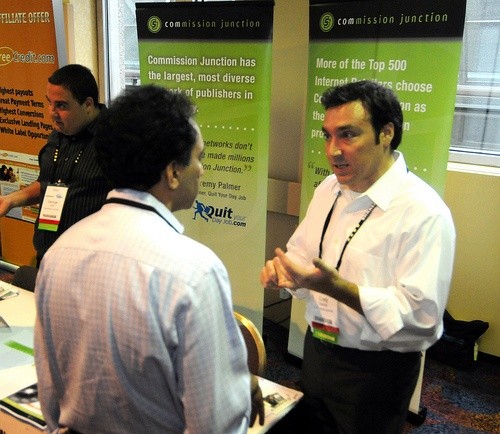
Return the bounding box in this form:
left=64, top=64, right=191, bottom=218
left=261, top=78, right=458, bottom=434
left=30, top=81, right=277, bottom=433
left=0, top=64, right=119, bottom=292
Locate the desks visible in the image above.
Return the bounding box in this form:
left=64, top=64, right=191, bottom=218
left=0, top=279, right=303, bottom=434
